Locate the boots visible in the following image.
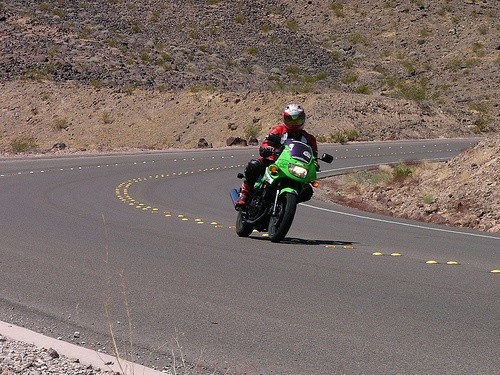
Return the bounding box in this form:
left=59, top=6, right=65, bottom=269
left=234, top=180, right=250, bottom=206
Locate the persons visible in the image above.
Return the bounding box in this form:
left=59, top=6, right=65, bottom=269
left=235, top=104, right=320, bottom=210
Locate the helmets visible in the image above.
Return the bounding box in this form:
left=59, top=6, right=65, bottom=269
left=283, top=104, right=306, bottom=132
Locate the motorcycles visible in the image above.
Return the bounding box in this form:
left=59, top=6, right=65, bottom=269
left=227, top=138, right=335, bottom=243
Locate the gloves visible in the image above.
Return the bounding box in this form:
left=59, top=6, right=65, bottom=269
left=259, top=146, right=275, bottom=158
